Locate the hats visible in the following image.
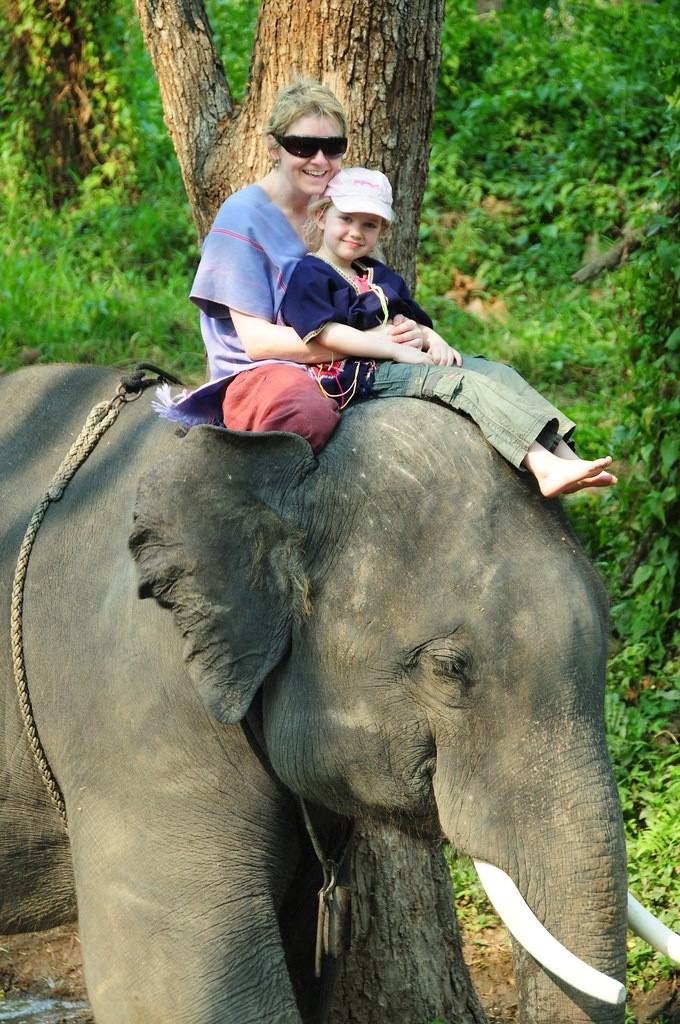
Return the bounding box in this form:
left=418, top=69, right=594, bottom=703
left=319, top=167, right=393, bottom=221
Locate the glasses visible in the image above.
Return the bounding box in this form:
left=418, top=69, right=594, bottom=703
left=268, top=131, right=347, bottom=159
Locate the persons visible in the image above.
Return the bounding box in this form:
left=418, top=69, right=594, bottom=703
left=188, top=78, right=620, bottom=500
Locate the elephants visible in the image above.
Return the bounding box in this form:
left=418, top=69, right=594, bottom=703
left=0, top=358, right=680, bottom=1024
